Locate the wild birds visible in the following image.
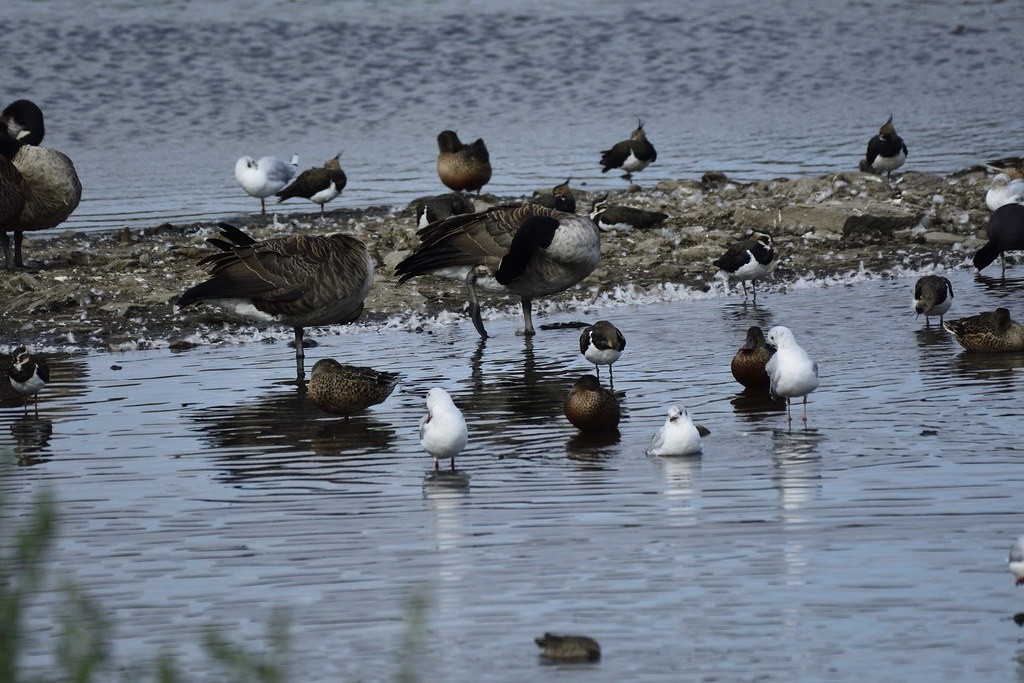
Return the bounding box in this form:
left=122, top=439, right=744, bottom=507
left=306, top=358, right=402, bottom=416
left=235, top=152, right=348, bottom=218
left=647, top=405, right=704, bottom=458
left=859, top=114, right=909, bottom=178
left=0, top=100, right=82, bottom=271
left=7, top=346, right=50, bottom=417
left=416, top=195, right=475, bottom=231
left=564, top=374, right=620, bottom=431
left=394, top=178, right=601, bottom=337
left=175, top=221, right=374, bottom=384
left=730, top=326, right=777, bottom=390
left=765, top=326, right=820, bottom=406
left=943, top=308, right=1024, bottom=353
left=418, top=388, right=468, bottom=474
left=908, top=275, right=955, bottom=325
left=436, top=130, right=492, bottom=194
left=537, top=118, right=667, bottom=233
left=972, top=175, right=1024, bottom=273
left=712, top=238, right=776, bottom=299
left=577, top=321, right=626, bottom=374
